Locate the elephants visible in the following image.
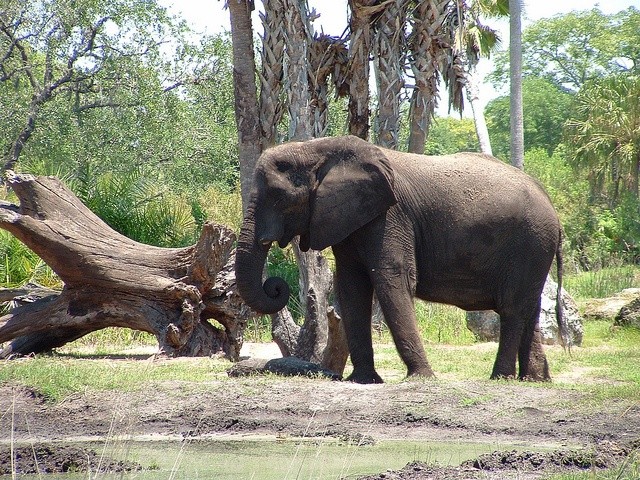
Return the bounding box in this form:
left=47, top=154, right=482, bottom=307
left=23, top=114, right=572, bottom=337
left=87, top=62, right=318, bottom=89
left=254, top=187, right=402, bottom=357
left=233, top=134, right=574, bottom=384
